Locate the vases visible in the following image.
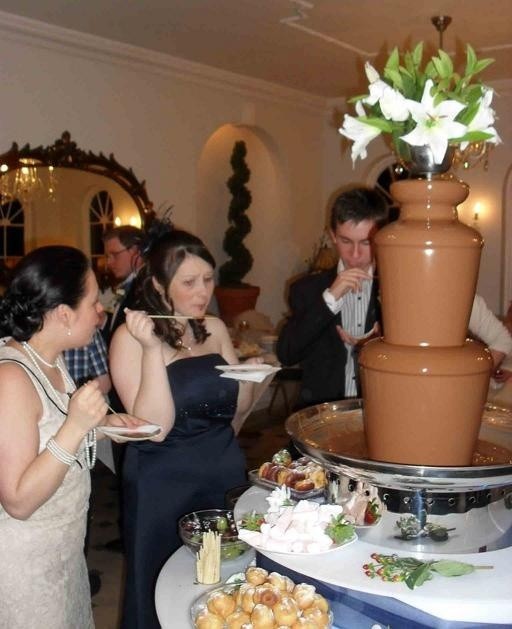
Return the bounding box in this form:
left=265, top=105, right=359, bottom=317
left=396, top=132, right=460, bottom=179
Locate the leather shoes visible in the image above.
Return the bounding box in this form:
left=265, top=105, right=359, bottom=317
left=105, top=537, right=125, bottom=551
left=88, top=573, right=101, bottom=595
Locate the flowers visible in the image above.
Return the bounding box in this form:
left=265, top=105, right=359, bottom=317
left=336, top=41, right=501, bottom=166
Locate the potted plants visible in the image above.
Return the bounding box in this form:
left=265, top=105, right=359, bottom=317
left=213, top=139, right=261, bottom=326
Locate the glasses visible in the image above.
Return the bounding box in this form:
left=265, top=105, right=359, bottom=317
left=103, top=247, right=128, bottom=263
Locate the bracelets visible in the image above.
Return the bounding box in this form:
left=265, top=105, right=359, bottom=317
left=46, top=437, right=76, bottom=465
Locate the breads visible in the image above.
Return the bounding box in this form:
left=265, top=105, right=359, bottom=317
left=257, top=457, right=315, bottom=491
left=196, top=566, right=329, bottom=629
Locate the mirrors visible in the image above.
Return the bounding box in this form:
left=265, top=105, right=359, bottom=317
left=0, top=126, right=157, bottom=292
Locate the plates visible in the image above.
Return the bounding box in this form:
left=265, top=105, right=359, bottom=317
left=96, top=423, right=163, bottom=443
left=347, top=504, right=386, bottom=528
left=247, top=468, right=326, bottom=500
left=216, top=363, right=272, bottom=373
left=238, top=516, right=358, bottom=558
left=188, top=583, right=334, bottom=629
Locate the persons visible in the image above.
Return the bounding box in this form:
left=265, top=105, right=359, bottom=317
left=0, top=244, right=153, bottom=629
left=103, top=224, right=149, bottom=551
left=276, top=186, right=382, bottom=460
left=61, top=329, right=110, bottom=597
left=110, top=229, right=266, bottom=629
left=466, top=296, right=511, bottom=372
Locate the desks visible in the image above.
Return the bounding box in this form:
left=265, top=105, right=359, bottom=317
left=154, top=473, right=510, bottom=629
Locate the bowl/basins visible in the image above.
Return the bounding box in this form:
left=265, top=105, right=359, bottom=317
left=178, top=509, right=254, bottom=562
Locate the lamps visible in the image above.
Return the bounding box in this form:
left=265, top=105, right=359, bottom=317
left=0, top=158, right=56, bottom=205
left=428, top=12, right=493, bottom=173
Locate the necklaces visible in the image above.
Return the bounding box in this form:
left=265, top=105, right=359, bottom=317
left=20, top=339, right=98, bottom=471
left=169, top=329, right=197, bottom=353
